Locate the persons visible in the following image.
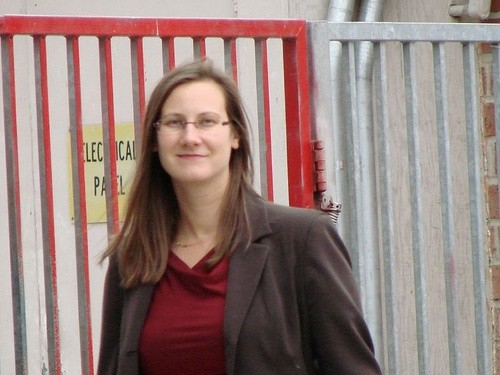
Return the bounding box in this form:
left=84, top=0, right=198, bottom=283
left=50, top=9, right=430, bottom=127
left=94, top=57, right=384, bottom=374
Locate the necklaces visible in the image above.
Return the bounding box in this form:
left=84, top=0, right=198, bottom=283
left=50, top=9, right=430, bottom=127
left=171, top=238, right=221, bottom=247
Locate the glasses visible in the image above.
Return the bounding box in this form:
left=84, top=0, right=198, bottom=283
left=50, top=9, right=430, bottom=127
left=152, top=114, right=232, bottom=130
